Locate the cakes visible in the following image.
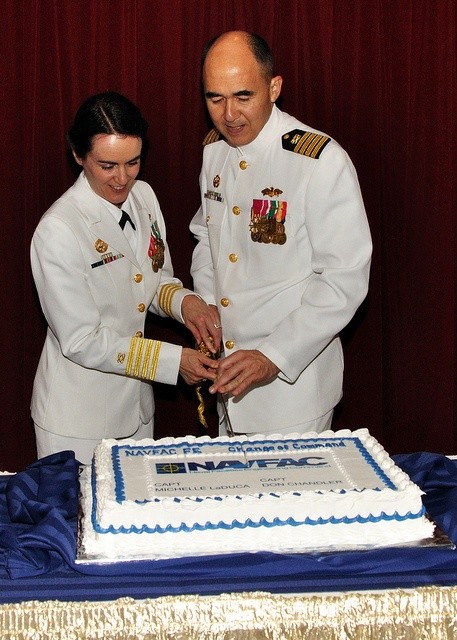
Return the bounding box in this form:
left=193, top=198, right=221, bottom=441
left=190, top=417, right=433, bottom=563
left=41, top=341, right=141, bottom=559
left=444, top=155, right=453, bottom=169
left=80, top=427, right=437, bottom=554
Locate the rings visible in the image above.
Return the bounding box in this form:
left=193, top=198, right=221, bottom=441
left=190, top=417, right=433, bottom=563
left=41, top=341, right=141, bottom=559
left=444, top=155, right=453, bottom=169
left=213, top=323, right=221, bottom=329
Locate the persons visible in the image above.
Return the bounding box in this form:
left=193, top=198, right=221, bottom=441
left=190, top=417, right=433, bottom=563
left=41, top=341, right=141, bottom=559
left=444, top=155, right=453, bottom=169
left=30, top=92, right=222, bottom=466
left=189, top=30, right=373, bottom=437
